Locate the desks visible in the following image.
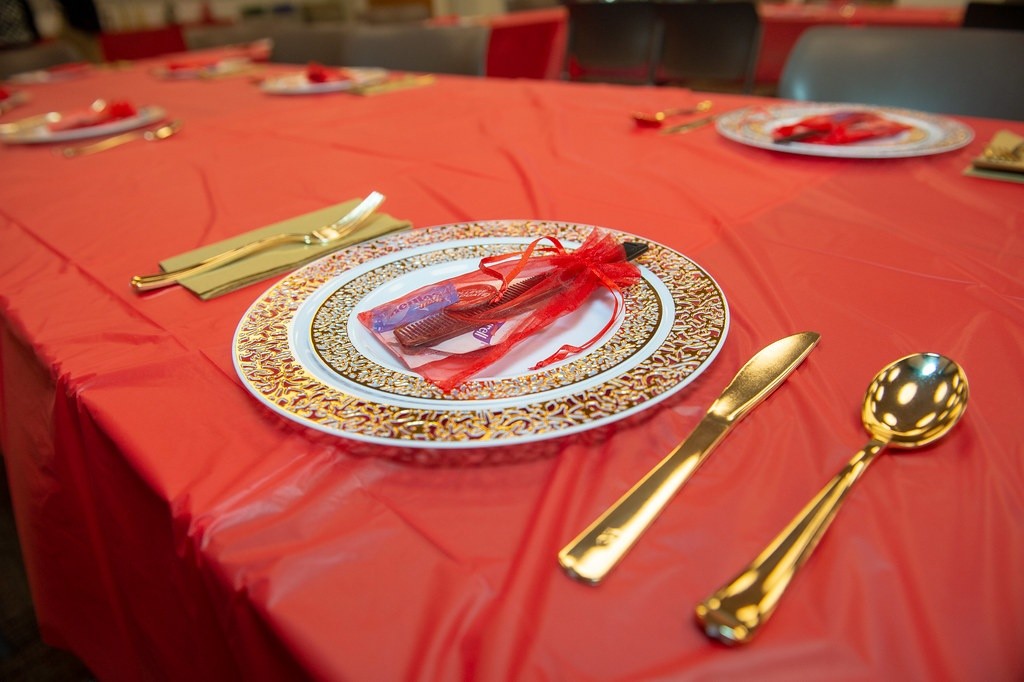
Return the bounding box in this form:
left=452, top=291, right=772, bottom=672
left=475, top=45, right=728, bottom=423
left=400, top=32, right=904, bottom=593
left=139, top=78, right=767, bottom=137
left=428, top=8, right=568, bottom=79
left=0, top=44, right=1023, bottom=682
left=757, top=0, right=964, bottom=87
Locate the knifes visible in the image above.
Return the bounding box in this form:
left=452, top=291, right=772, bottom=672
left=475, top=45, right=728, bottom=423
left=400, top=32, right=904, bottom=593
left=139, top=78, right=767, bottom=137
left=558, top=330, right=820, bottom=584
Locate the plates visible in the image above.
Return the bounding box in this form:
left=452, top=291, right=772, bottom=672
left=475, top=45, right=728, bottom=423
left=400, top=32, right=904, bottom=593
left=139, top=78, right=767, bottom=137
left=0, top=107, right=165, bottom=142
left=231, top=220, right=730, bottom=447
left=715, top=103, right=976, bottom=158
left=261, top=68, right=384, bottom=95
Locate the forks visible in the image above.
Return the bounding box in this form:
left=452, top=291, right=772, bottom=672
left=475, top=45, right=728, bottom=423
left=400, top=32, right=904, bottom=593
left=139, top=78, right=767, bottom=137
left=131, top=191, right=386, bottom=291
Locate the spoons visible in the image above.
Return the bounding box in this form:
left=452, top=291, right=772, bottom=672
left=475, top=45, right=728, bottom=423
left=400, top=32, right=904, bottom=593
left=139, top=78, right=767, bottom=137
left=65, top=118, right=185, bottom=157
left=694, top=351, right=970, bottom=646
